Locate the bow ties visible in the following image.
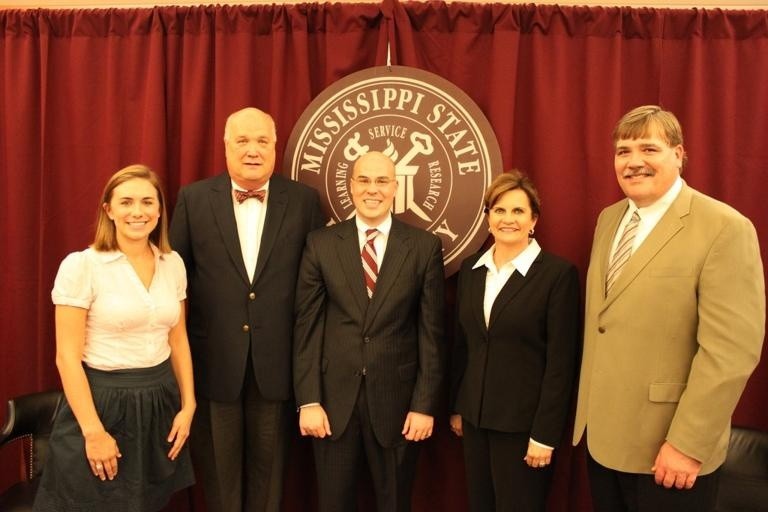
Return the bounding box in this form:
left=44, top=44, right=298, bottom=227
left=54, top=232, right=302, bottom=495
left=234, top=190, right=266, bottom=206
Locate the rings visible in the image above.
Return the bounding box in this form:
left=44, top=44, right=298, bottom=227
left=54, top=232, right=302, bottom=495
left=96, top=464, right=103, bottom=470
left=540, top=463, right=545, bottom=468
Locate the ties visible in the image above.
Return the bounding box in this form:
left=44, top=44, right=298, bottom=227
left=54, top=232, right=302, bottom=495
left=361, top=229, right=380, bottom=301
left=605, top=210, right=641, bottom=300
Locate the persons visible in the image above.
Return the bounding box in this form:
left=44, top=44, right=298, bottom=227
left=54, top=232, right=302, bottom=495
left=168, top=107, right=324, bottom=512
left=572, top=105, right=766, bottom=512
left=292, top=151, right=446, bottom=512
left=31, top=164, right=197, bottom=512
left=447, top=168, right=581, bottom=512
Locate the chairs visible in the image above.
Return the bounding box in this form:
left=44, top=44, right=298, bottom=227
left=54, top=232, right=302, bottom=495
left=0, top=390, right=63, bottom=511
